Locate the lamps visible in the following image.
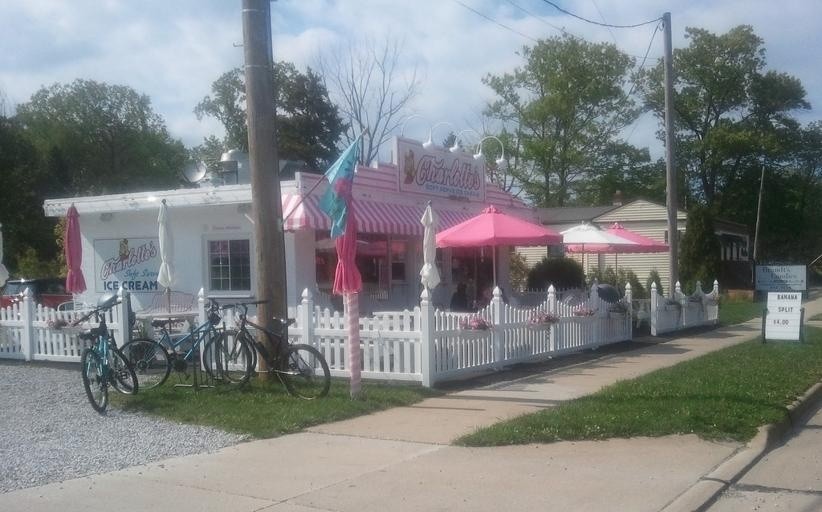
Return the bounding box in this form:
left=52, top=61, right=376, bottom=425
left=401, top=115, right=508, bottom=171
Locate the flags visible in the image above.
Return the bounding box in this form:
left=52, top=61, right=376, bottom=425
left=324, top=130, right=367, bottom=240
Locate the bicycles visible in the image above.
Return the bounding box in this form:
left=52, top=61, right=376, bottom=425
left=113, top=298, right=258, bottom=391
left=202, top=298, right=332, bottom=401
left=74, top=293, right=138, bottom=414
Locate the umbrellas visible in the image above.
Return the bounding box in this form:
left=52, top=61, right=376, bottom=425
left=554, top=218, right=642, bottom=275
left=63, top=201, right=89, bottom=311
left=155, top=199, right=179, bottom=334
left=417, top=200, right=440, bottom=308
left=435, top=201, right=566, bottom=289
left=331, top=196, right=364, bottom=303
left=562, top=221, right=672, bottom=289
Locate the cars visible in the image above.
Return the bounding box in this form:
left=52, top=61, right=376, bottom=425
left=0, top=276, right=74, bottom=311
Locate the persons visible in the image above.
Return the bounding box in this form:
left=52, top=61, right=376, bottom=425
left=448, top=279, right=473, bottom=314
left=499, top=287, right=510, bottom=305
left=477, top=286, right=493, bottom=310
